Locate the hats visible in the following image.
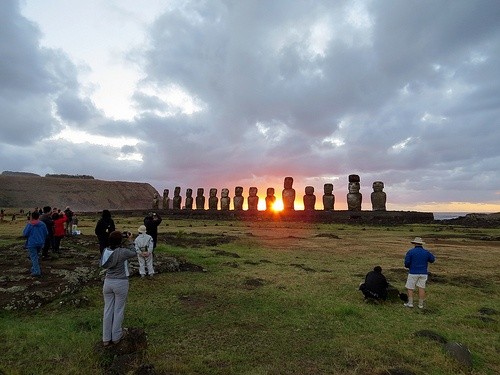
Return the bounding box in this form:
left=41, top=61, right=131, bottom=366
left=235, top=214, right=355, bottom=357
left=411, top=237, right=425, bottom=245
left=102, top=210, right=111, bottom=218
left=138, top=225, right=147, bottom=233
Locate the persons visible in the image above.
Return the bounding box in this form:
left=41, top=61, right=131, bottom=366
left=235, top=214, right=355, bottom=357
left=143, top=211, right=162, bottom=252
left=9, top=206, right=79, bottom=258
left=404, top=237, right=436, bottom=309
left=359, top=266, right=388, bottom=303
left=24, top=212, right=48, bottom=277
left=100, top=231, right=137, bottom=346
left=95, top=209, right=115, bottom=256
left=135, top=225, right=153, bottom=279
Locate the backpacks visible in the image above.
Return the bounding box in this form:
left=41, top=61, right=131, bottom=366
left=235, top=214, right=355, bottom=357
left=152, top=213, right=158, bottom=222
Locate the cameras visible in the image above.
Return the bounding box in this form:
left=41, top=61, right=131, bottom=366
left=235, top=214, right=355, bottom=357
left=121, top=232, right=131, bottom=238
left=153, top=212, right=157, bottom=216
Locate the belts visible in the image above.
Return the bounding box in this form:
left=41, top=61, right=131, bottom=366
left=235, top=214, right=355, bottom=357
left=366, top=289, right=377, bottom=296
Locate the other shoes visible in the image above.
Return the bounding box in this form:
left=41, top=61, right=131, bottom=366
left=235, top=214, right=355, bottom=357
left=374, top=299, right=379, bottom=305
left=31, top=274, right=41, bottom=278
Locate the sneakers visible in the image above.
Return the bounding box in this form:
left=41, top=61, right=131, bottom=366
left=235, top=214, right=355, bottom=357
left=404, top=303, right=413, bottom=307
left=103, top=342, right=109, bottom=346
left=150, top=274, right=153, bottom=277
left=114, top=327, right=128, bottom=344
left=418, top=305, right=423, bottom=308
left=141, top=274, right=145, bottom=277
